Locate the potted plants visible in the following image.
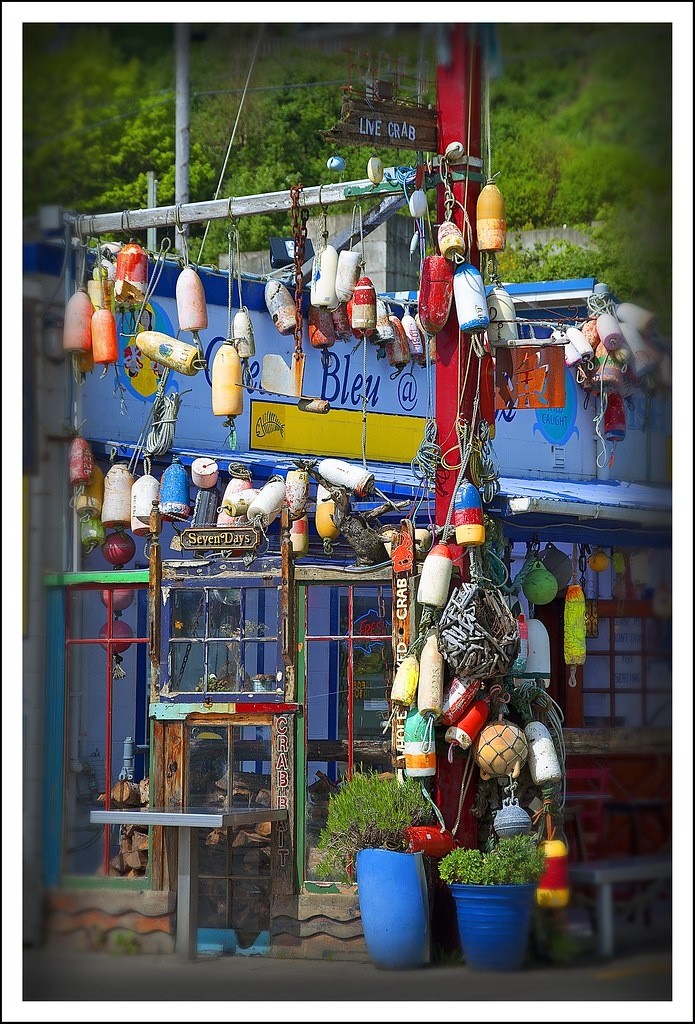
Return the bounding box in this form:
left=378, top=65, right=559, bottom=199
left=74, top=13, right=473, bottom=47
left=316, top=761, right=435, bottom=970
left=438, top=830, right=550, bottom=970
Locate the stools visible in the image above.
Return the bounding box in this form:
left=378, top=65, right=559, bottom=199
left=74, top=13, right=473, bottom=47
left=567, top=854, right=671, bottom=957
left=604, top=799, right=668, bottom=926
left=564, top=803, right=600, bottom=932
